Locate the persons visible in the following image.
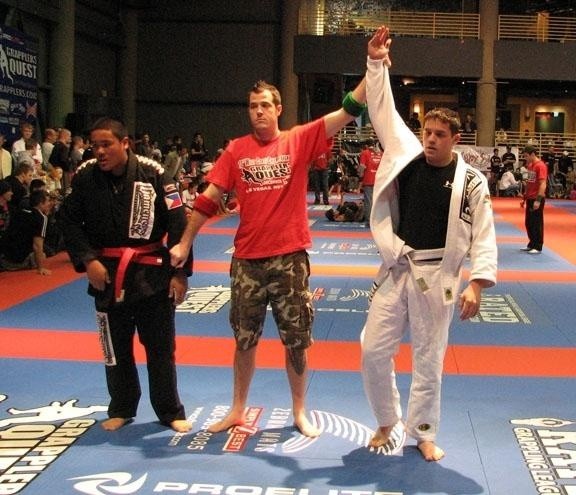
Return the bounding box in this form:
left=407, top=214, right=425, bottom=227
left=168, top=53, right=394, bottom=438
left=519, top=145, right=549, bottom=254
left=313, top=119, right=383, bottom=228
left=406, top=112, right=422, bottom=135
left=128, top=130, right=240, bottom=221
left=460, top=114, right=479, bottom=144
left=0, top=121, right=98, bottom=276
left=358, top=26, right=499, bottom=462
left=54, top=118, right=195, bottom=433
left=490, top=127, right=576, bottom=199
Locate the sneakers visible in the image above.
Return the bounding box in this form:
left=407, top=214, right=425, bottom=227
left=520, top=247, right=541, bottom=253
